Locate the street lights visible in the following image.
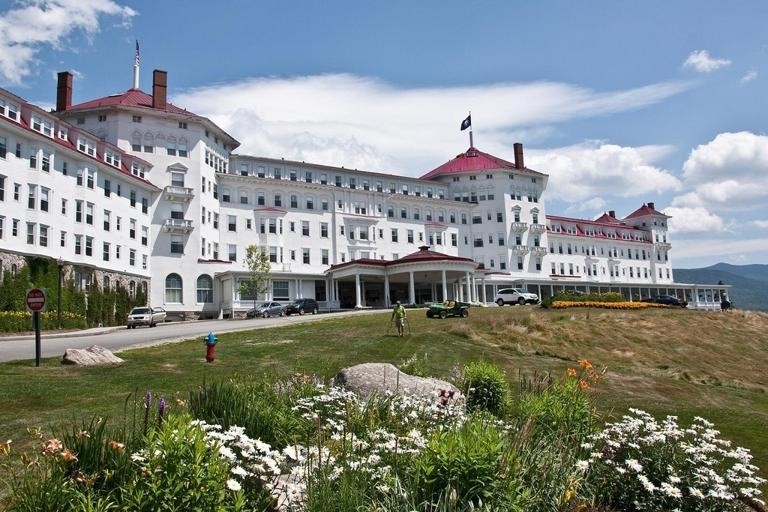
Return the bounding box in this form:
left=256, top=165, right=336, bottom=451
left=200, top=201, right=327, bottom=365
left=56, top=256, right=66, bottom=330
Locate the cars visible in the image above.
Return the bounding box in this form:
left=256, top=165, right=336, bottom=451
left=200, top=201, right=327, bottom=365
left=247, top=298, right=319, bottom=317
left=640, top=295, right=685, bottom=307
left=495, top=288, right=539, bottom=306
left=126, top=305, right=167, bottom=328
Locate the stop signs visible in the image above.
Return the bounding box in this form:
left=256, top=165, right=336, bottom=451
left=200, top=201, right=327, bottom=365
left=26, top=288, right=46, bottom=311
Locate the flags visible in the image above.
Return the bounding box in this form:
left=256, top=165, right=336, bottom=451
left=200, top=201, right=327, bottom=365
left=460, top=115, right=471, bottom=131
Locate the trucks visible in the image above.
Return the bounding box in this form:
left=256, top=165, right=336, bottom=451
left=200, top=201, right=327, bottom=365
left=426, top=299, right=470, bottom=318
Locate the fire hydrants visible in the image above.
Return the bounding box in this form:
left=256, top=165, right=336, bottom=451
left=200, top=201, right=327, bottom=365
left=203, top=330, right=218, bottom=363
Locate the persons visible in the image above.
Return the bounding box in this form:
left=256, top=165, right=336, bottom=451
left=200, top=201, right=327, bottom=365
left=391, top=300, right=406, bottom=337
